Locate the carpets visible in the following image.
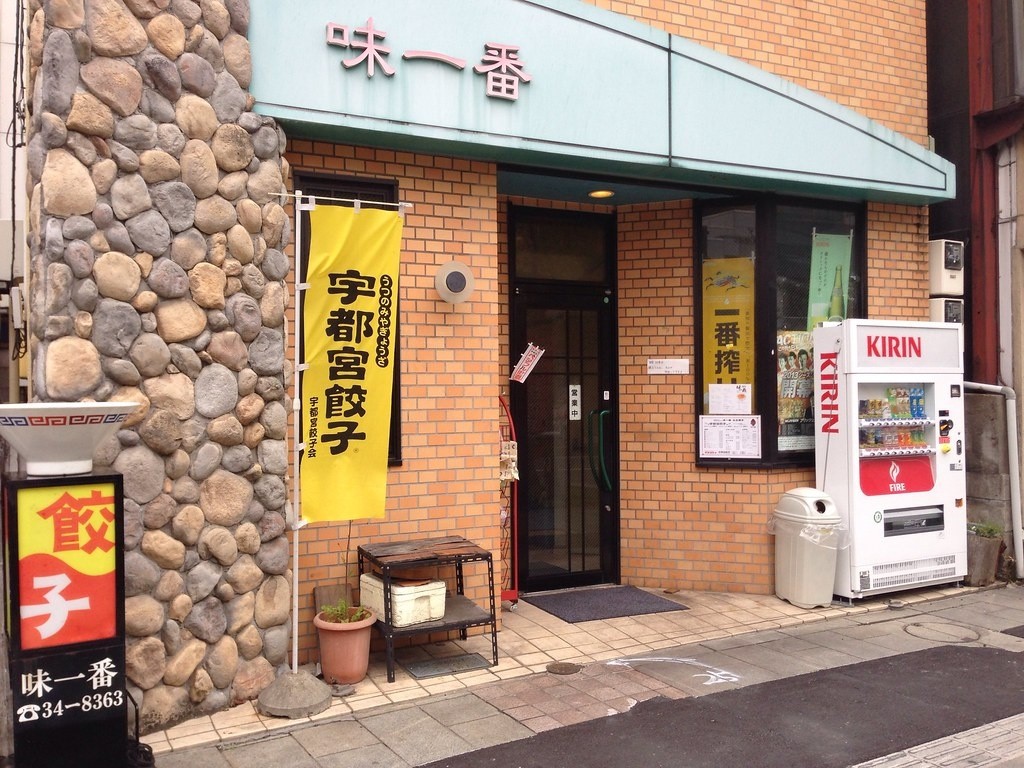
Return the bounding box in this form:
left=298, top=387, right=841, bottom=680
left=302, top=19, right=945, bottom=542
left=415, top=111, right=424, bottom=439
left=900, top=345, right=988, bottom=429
left=520, top=584, right=689, bottom=624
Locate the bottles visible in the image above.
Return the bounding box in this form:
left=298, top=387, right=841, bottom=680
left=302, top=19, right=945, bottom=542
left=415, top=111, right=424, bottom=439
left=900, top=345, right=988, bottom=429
left=884, top=427, right=924, bottom=444
left=828, top=265, right=844, bottom=321
left=889, top=395, right=910, bottom=418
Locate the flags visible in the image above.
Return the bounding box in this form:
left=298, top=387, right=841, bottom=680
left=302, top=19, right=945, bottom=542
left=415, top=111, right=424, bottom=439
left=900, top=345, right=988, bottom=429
left=301, top=197, right=404, bottom=523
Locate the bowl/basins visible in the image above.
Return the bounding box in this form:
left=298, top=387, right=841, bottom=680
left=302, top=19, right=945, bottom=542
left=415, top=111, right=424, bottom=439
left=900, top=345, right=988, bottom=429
left=0, top=403, right=139, bottom=475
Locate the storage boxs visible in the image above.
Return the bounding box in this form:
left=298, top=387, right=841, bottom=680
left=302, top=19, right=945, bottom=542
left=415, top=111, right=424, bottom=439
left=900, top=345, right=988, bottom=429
left=360, top=572, right=447, bottom=628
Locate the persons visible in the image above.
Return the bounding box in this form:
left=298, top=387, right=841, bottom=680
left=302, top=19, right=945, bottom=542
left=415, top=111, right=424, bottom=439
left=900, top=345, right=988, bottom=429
left=778, top=348, right=813, bottom=419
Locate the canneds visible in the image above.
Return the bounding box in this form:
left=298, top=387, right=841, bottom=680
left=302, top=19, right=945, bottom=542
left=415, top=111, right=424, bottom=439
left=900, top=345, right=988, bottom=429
left=858, top=398, right=884, bottom=445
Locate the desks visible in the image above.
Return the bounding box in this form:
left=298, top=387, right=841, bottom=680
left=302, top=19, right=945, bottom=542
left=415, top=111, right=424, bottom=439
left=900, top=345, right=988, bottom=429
left=357, top=535, right=498, bottom=682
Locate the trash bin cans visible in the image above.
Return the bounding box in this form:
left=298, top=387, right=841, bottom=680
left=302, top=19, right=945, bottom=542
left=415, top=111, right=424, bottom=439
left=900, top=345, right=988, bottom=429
left=773, top=486, right=843, bottom=608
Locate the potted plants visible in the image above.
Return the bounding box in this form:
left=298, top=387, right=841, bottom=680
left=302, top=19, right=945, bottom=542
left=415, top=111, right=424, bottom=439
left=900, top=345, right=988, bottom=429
left=313, top=520, right=376, bottom=686
